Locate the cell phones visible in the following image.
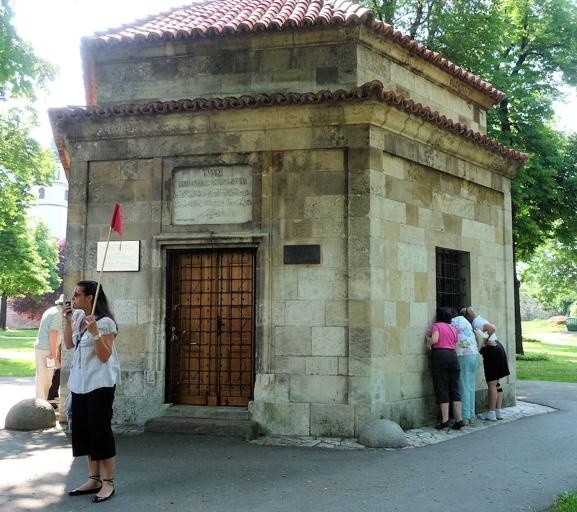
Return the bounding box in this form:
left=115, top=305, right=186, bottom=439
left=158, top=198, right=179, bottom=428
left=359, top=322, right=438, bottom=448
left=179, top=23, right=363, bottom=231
left=65, top=302, right=72, bottom=315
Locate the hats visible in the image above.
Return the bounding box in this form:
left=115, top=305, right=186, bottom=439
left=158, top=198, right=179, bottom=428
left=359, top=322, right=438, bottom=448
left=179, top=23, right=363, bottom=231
left=54, top=293, right=64, bottom=304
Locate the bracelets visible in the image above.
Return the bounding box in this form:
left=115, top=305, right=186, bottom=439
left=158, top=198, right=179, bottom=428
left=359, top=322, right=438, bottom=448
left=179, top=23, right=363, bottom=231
left=92, top=332, right=100, bottom=341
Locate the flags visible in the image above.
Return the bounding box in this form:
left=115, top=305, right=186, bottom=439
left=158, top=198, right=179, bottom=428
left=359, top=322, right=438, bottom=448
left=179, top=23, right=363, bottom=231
left=113, top=203, right=121, bottom=235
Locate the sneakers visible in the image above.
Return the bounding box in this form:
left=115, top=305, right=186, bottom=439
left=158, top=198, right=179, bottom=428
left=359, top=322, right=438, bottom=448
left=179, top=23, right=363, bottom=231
left=477, top=409, right=503, bottom=421
left=452, top=419, right=463, bottom=429
left=463, top=419, right=476, bottom=425
left=434, top=420, right=450, bottom=429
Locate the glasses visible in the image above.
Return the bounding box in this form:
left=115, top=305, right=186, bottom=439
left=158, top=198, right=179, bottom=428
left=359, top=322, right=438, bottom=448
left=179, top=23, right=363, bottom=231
left=73, top=292, right=85, bottom=297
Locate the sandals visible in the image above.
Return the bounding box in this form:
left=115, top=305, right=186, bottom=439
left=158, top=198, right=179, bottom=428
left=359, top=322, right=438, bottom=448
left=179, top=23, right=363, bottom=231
left=68, top=475, right=102, bottom=495
left=92, top=478, right=115, bottom=503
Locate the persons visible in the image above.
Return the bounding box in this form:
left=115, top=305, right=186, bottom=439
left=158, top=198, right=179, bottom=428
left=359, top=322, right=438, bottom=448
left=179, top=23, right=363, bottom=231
left=46, top=344, right=61, bottom=409
left=443, top=306, right=480, bottom=425
left=459, top=305, right=510, bottom=422
left=32, top=294, right=64, bottom=409
left=425, top=307, right=464, bottom=431
left=61, top=280, right=121, bottom=503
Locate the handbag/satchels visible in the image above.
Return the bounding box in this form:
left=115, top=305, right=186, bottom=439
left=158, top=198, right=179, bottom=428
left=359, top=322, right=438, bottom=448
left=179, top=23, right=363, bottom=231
left=474, top=328, right=489, bottom=351
